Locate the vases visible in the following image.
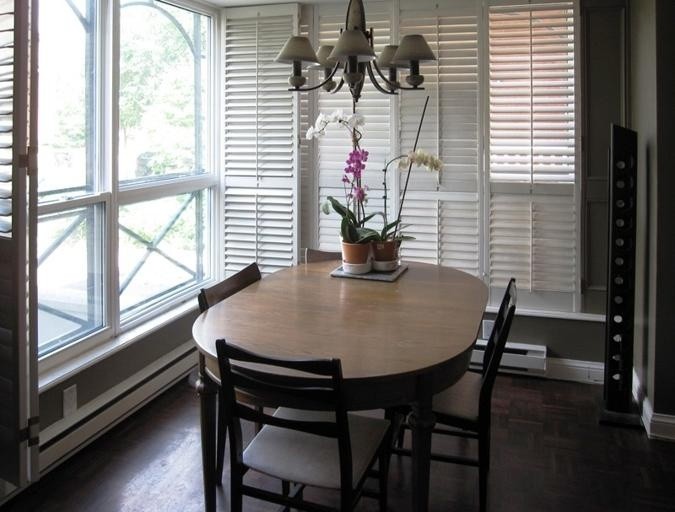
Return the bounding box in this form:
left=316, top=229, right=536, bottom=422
left=342, top=239, right=403, bottom=273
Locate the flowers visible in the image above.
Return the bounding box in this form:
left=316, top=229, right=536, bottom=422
left=303, top=94, right=446, bottom=240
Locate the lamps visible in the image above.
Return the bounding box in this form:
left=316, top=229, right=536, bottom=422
left=274, top=0, right=438, bottom=105
